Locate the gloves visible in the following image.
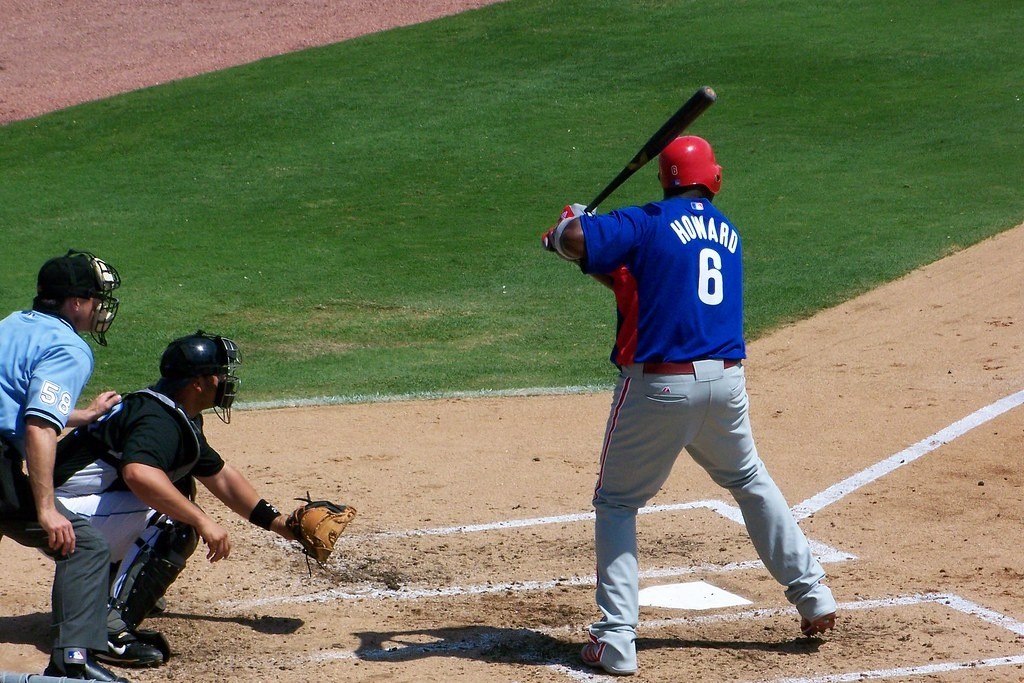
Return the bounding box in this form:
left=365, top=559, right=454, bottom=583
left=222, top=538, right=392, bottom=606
left=553, top=202, right=598, bottom=259
left=541, top=229, right=557, bottom=252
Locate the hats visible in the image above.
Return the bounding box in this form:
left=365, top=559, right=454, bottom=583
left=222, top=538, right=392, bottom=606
left=37, top=254, right=101, bottom=300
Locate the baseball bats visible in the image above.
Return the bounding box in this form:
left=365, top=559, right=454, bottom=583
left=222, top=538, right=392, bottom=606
left=547, top=85, right=718, bottom=253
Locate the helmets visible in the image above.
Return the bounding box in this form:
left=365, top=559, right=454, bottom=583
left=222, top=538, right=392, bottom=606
left=659, top=135, right=722, bottom=194
left=160, top=335, right=227, bottom=380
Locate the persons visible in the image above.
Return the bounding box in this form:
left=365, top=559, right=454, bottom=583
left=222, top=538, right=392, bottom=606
left=0, top=249, right=130, bottom=683
left=542, top=135, right=838, bottom=675
left=36, top=329, right=298, bottom=667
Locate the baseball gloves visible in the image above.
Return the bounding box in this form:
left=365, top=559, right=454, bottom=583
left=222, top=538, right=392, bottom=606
left=287, top=490, right=358, bottom=579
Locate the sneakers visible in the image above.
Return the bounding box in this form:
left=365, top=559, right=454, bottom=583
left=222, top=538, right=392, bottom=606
left=43, top=648, right=129, bottom=682
left=581, top=643, right=638, bottom=674
left=802, top=609, right=835, bottom=635
left=88, top=629, right=163, bottom=665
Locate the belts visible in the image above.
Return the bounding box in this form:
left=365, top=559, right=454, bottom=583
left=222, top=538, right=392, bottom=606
left=642, top=356, right=742, bottom=374
